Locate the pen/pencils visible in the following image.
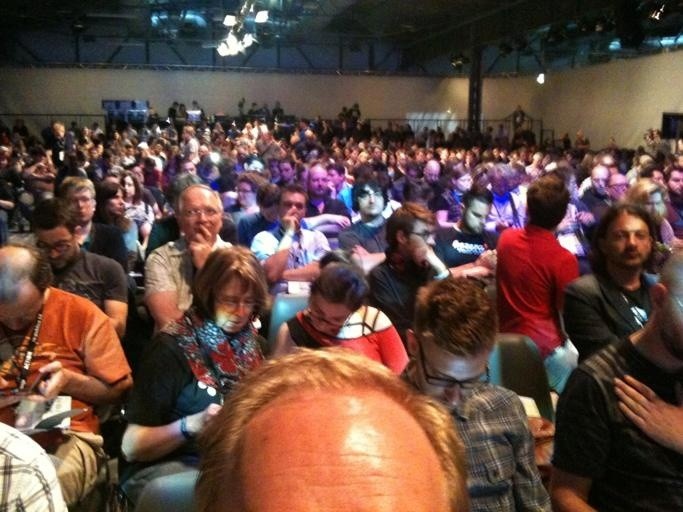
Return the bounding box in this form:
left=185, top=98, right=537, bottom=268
left=219, top=393, right=224, bottom=405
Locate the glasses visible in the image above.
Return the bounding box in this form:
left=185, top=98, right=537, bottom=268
left=305, top=305, right=351, bottom=328
left=214, top=296, right=259, bottom=313
left=410, top=229, right=433, bottom=239
left=284, top=201, right=308, bottom=210
left=177, top=208, right=220, bottom=218
left=418, top=343, right=490, bottom=389
left=36, top=238, right=74, bottom=254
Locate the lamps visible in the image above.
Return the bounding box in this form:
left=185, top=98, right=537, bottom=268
left=524, top=41, right=547, bottom=84
left=218, top=1, right=269, bottom=56
left=650, top=4, right=665, bottom=21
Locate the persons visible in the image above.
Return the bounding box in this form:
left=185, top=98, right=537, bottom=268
left=273, top=260, right=410, bottom=378
left=550, top=250, right=683, bottom=512
left=496, top=176, right=580, bottom=396
left=195, top=346, right=471, bottom=512
left=144, top=185, right=234, bottom=344
left=121, top=245, right=272, bottom=512
left=1, top=97, right=683, bottom=296
left=32, top=197, right=130, bottom=338
left=365, top=202, right=453, bottom=355
left=1, top=245, right=134, bottom=509
left=562, top=204, right=659, bottom=367
left=0, top=421, right=68, bottom=512
left=398, top=277, right=553, bottom=512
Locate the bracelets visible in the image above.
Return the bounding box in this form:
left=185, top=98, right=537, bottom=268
left=181, top=414, right=196, bottom=441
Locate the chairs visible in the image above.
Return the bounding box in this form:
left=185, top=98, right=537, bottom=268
left=268, top=293, right=309, bottom=348
left=119, top=462, right=208, bottom=512
left=479, top=333, right=554, bottom=420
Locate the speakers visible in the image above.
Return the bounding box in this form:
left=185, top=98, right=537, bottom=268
left=662, top=112, right=683, bottom=139
